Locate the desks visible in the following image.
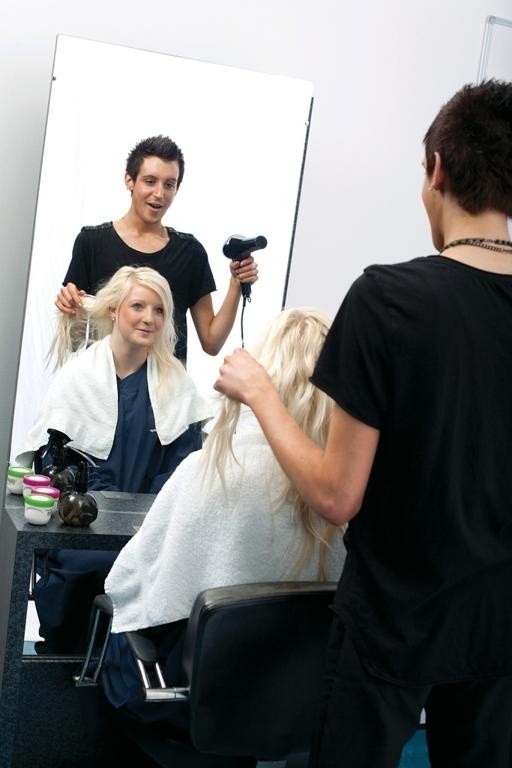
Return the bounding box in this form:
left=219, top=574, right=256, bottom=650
left=4, top=489, right=159, bottom=508
left=2, top=509, right=144, bottom=766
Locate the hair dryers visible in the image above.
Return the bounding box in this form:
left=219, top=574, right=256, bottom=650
left=221, top=234, right=268, bottom=299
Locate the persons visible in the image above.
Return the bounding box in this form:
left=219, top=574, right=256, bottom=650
left=96, top=304, right=348, bottom=717
left=34, top=263, right=216, bottom=664
left=55, top=132, right=259, bottom=355
left=212, top=78, right=511, bottom=766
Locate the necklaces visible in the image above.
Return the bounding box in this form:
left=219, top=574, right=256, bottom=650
left=439, top=238, right=510, bottom=256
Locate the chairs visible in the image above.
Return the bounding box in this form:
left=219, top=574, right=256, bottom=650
left=71, top=579, right=344, bottom=766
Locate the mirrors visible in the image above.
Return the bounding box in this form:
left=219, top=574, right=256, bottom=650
left=7, top=32, right=310, bottom=508
